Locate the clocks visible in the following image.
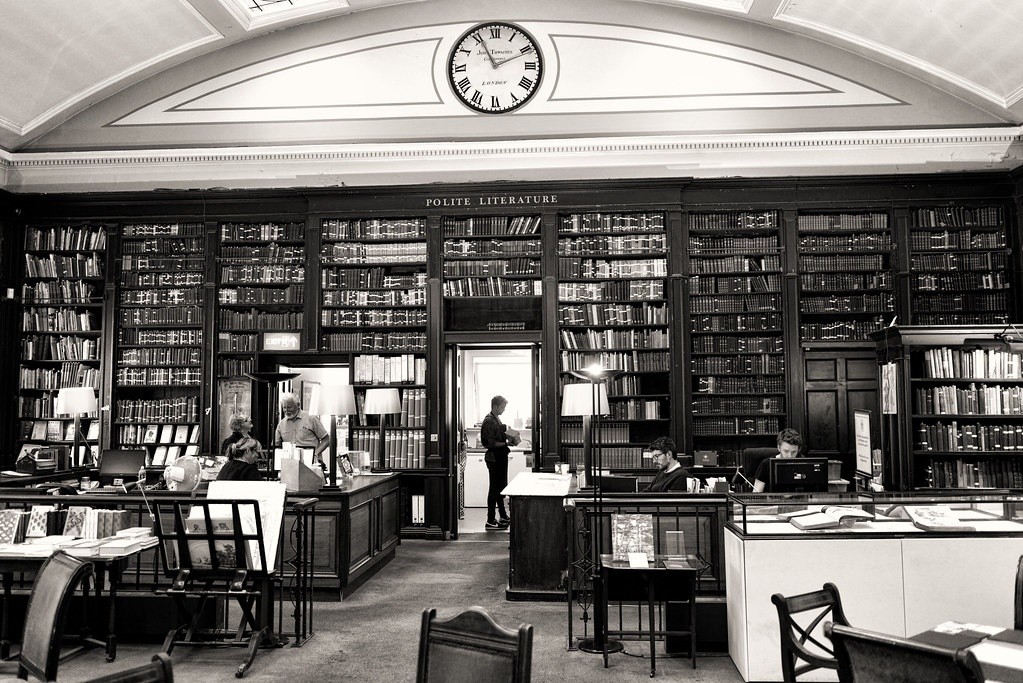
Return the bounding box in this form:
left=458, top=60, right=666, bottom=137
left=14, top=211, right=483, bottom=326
left=448, top=22, right=543, bottom=114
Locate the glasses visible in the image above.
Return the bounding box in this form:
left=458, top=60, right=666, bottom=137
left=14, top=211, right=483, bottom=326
left=653, top=450, right=667, bottom=460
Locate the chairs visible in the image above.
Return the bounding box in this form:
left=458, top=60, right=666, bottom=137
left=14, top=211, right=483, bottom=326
left=824, top=621, right=985, bottom=683
left=744, top=447, right=780, bottom=493
left=415, top=606, right=534, bottom=683
left=151, top=497, right=288, bottom=678
left=0, top=549, right=96, bottom=683
left=1013, top=555, right=1023, bottom=632
left=771, top=582, right=855, bottom=683
left=85, top=651, right=174, bottom=683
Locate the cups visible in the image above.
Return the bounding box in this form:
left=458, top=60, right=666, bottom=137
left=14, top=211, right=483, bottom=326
left=561, top=463, right=570, bottom=475
left=555, top=463, right=563, bottom=474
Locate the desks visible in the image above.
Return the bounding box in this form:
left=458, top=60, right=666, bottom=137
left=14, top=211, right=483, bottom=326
left=0, top=536, right=160, bottom=664
left=600, top=553, right=703, bottom=679
left=464, top=428, right=532, bottom=508
left=907, top=621, right=1023, bottom=683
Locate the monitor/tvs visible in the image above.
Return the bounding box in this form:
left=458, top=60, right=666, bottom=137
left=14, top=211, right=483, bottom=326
left=770, top=457, right=828, bottom=493
left=98, top=449, right=146, bottom=484
left=599, top=475, right=638, bottom=493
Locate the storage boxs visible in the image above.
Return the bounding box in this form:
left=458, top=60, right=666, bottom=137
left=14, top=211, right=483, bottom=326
left=143, top=425, right=158, bottom=444
left=827, top=459, right=843, bottom=481
left=31, top=421, right=48, bottom=441
left=160, top=425, right=174, bottom=444
left=694, top=451, right=718, bottom=467
left=504, top=428, right=522, bottom=447
left=79, top=445, right=99, bottom=468
left=112, top=478, right=124, bottom=486
left=49, top=445, right=69, bottom=470
left=174, top=426, right=188, bottom=443
left=47, top=421, right=64, bottom=441
left=164, top=446, right=181, bottom=466
left=189, top=425, right=200, bottom=443
left=713, top=482, right=729, bottom=493
left=86, top=419, right=99, bottom=440
left=152, top=446, right=167, bottom=466
left=185, top=445, right=200, bottom=456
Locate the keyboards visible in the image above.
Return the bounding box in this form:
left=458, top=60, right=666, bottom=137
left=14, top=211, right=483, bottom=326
left=86, top=487, right=118, bottom=494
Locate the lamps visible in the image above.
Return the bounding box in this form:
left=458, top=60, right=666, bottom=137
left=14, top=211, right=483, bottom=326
left=163, top=454, right=203, bottom=492
left=308, top=384, right=357, bottom=494
left=362, top=388, right=402, bottom=473
left=55, top=387, right=97, bottom=468
left=560, top=382, right=611, bottom=489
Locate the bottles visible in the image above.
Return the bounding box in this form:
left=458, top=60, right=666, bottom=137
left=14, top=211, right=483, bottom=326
left=138, top=466, right=146, bottom=489
left=81, top=477, right=91, bottom=490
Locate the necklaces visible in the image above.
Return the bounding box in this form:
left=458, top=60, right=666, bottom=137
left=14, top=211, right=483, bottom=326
left=247, top=434, right=250, bottom=437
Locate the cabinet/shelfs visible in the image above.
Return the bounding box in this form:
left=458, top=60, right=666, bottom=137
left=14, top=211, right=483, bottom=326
left=0, top=171, right=1023, bottom=543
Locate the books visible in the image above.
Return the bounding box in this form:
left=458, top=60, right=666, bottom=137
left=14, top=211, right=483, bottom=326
left=443, top=215, right=542, bottom=296
left=776, top=506, right=828, bottom=521
left=0, top=218, right=429, bottom=557
left=875, top=505, right=903, bottom=517
left=908, top=620, right=1023, bottom=652
left=902, top=506, right=976, bottom=531
left=557, top=204, right=1023, bottom=492
left=790, top=506, right=875, bottom=530
left=611, top=513, right=654, bottom=562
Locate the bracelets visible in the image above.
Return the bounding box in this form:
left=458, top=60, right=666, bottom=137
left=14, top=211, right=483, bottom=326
left=315, top=454, right=318, bottom=457
left=505, top=443, right=507, bottom=447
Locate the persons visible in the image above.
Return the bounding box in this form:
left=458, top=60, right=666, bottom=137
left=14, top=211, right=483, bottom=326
left=752, top=429, right=802, bottom=492
left=481, top=396, right=515, bottom=530
left=220, top=413, right=256, bottom=456
left=216, top=438, right=264, bottom=481
left=270, top=392, right=329, bottom=460
left=642, top=437, right=691, bottom=493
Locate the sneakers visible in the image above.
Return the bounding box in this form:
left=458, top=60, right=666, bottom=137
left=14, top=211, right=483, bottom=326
left=485, top=520, right=507, bottom=530
left=499, top=516, right=510, bottom=525
